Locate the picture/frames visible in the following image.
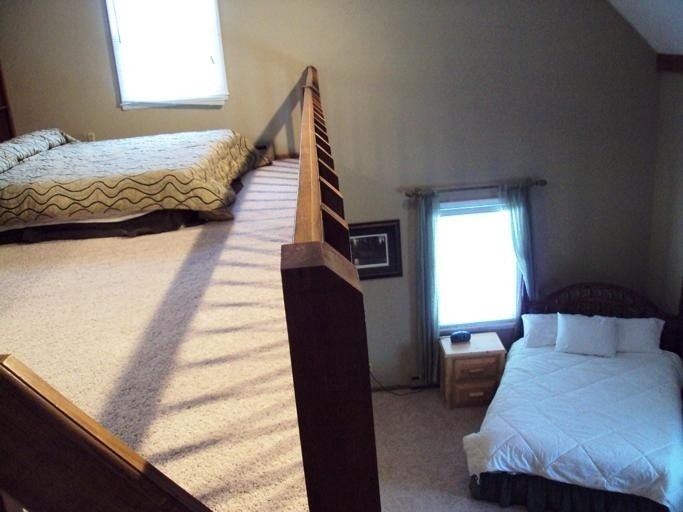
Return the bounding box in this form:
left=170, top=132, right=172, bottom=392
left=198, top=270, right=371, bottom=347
left=347, top=219, right=403, bottom=280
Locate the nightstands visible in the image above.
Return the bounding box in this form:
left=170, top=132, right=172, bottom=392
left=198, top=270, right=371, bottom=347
left=438, top=332, right=507, bottom=409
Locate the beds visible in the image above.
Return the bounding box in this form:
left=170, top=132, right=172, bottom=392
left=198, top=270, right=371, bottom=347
left=0, top=126, right=247, bottom=236
left=470, top=281, right=683, bottom=512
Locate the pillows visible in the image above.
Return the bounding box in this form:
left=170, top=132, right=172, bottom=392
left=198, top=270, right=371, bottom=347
left=520, top=311, right=665, bottom=358
